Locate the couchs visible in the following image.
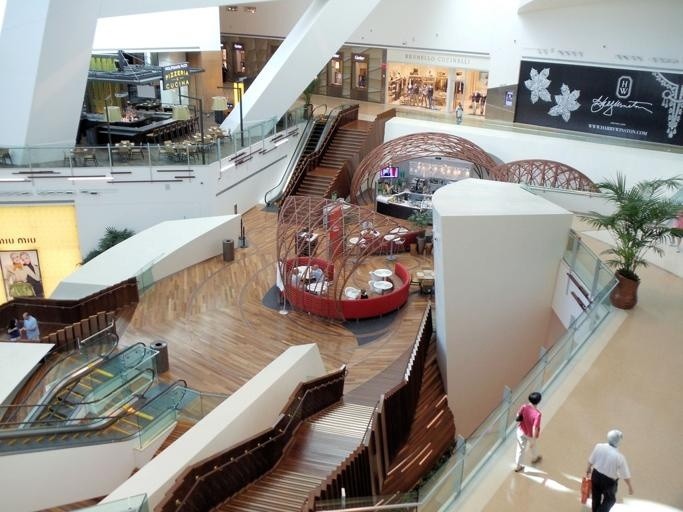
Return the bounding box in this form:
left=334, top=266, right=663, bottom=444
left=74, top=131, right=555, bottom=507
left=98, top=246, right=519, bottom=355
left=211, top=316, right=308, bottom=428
left=278, top=228, right=425, bottom=325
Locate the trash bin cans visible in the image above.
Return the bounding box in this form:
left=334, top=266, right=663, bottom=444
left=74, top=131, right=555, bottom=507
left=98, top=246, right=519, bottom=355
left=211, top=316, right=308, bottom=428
left=223, top=239, right=234, bottom=261
left=150, top=340, right=169, bottom=373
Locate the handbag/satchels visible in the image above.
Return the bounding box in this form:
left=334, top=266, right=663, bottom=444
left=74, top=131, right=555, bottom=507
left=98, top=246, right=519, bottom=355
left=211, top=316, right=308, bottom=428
left=579, top=475, right=593, bottom=505
left=516, top=414, right=524, bottom=422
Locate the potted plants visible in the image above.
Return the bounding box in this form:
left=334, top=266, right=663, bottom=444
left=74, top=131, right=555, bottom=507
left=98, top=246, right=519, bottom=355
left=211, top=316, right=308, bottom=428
left=576, top=170, right=683, bottom=311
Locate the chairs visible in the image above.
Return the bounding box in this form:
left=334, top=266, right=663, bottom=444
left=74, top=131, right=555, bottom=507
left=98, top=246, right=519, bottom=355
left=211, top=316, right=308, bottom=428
left=63, top=116, right=231, bottom=168
left=0, top=148, right=13, bottom=168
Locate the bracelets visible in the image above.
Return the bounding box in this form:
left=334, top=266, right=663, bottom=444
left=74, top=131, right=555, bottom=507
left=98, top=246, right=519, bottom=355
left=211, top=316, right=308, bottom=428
left=33, top=265, right=38, bottom=266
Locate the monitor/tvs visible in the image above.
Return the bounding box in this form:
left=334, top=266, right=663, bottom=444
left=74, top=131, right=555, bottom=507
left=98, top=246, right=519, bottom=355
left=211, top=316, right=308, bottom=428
left=380, top=167, right=399, bottom=178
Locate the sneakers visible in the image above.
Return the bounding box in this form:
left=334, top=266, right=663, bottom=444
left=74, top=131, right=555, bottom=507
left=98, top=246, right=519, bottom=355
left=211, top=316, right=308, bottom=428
left=514, top=464, right=525, bottom=472
left=531, top=456, right=542, bottom=464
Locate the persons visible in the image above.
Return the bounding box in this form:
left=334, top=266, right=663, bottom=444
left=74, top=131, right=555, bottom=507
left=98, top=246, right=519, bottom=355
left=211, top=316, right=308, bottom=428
left=474, top=93, right=480, bottom=115
left=419, top=83, right=428, bottom=109
left=425, top=85, right=433, bottom=109
left=455, top=102, right=464, bottom=124
left=5, top=251, right=45, bottom=298
left=383, top=182, right=392, bottom=196
left=3, top=252, right=40, bottom=299
left=410, top=83, right=420, bottom=107
left=18, top=311, right=40, bottom=340
left=471, top=92, right=476, bottom=115
left=310, top=263, right=324, bottom=282
left=6, top=318, right=21, bottom=342
left=480, top=93, right=486, bottom=115
left=511, top=391, right=544, bottom=472
left=354, top=289, right=368, bottom=300
left=288, top=267, right=305, bottom=289
left=382, top=168, right=390, bottom=176
left=584, top=428, right=634, bottom=512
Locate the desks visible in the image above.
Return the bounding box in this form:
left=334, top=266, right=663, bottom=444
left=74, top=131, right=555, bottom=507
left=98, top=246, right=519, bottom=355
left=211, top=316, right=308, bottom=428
left=418, top=266, right=434, bottom=299
